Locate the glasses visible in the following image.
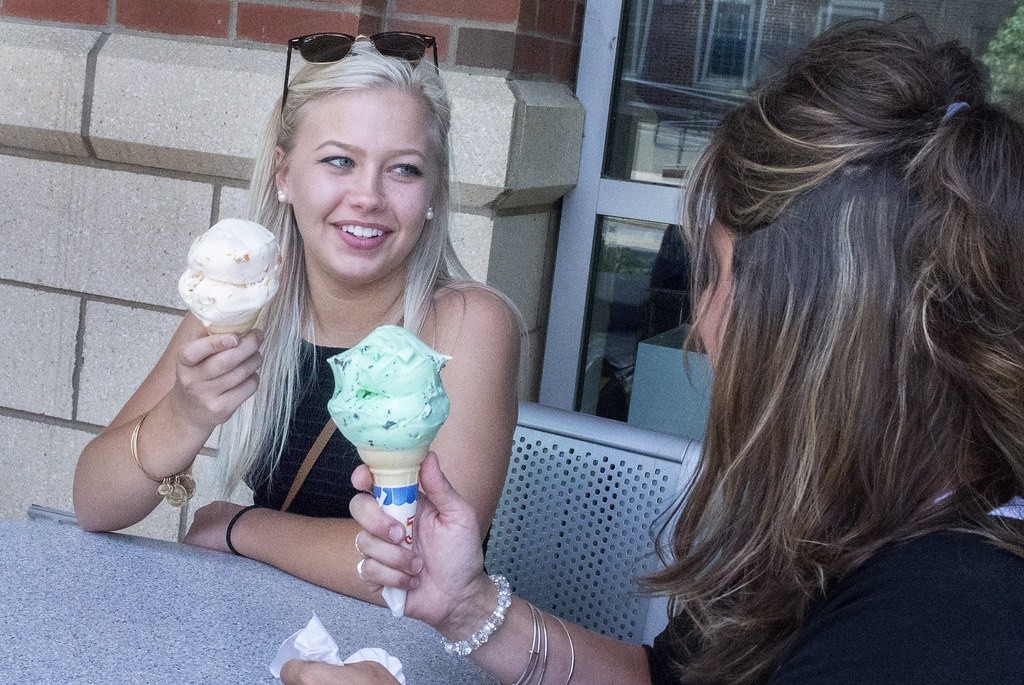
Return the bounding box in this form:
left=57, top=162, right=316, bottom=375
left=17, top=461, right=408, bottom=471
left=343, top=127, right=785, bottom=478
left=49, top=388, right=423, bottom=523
left=282, top=33, right=438, bottom=117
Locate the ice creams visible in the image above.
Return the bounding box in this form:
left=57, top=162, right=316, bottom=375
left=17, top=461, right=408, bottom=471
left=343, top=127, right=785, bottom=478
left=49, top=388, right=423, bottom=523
left=177, top=217, right=284, bottom=338
left=327, top=324, right=454, bottom=488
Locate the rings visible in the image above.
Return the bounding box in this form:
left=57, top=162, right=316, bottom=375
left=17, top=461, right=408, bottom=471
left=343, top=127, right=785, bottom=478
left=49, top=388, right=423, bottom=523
left=355, top=533, right=371, bottom=559
left=356, top=556, right=371, bottom=583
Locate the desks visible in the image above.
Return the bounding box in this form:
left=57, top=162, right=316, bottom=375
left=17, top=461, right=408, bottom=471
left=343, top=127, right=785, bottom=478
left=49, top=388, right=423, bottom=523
left=0, top=518, right=502, bottom=685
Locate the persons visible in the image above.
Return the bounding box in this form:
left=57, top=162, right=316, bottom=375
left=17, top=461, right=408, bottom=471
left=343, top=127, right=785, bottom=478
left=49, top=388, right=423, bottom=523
left=277, top=20, right=1024, bottom=684
left=76, top=29, right=521, bottom=617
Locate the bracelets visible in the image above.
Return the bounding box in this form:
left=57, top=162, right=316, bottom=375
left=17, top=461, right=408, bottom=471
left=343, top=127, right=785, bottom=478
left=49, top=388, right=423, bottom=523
left=441, top=574, right=513, bottom=658
left=131, top=412, right=197, bottom=507
left=510, top=598, right=575, bottom=685
left=226, top=505, right=266, bottom=557
